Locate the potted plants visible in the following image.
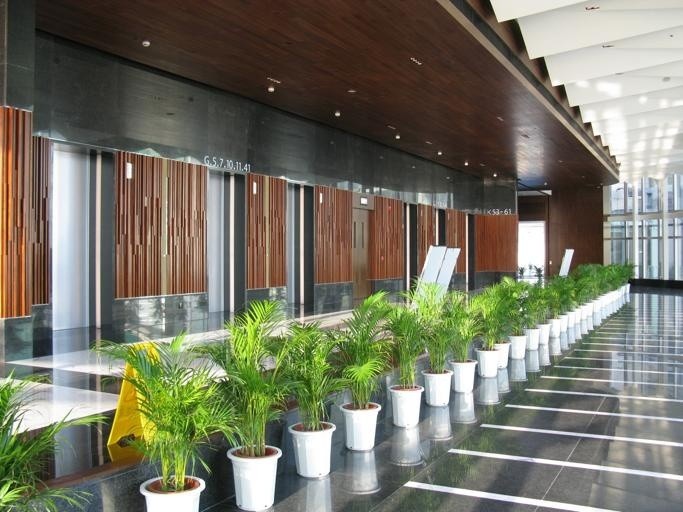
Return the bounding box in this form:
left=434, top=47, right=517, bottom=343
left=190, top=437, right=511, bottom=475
left=273, top=316, right=341, bottom=481
left=394, top=281, right=469, bottom=414
left=330, top=288, right=394, bottom=453
left=475, top=261, right=639, bottom=379
left=383, top=279, right=444, bottom=431
left=438, top=290, right=479, bottom=393
left=86, top=319, right=225, bottom=512
left=214, top=296, right=308, bottom=512
